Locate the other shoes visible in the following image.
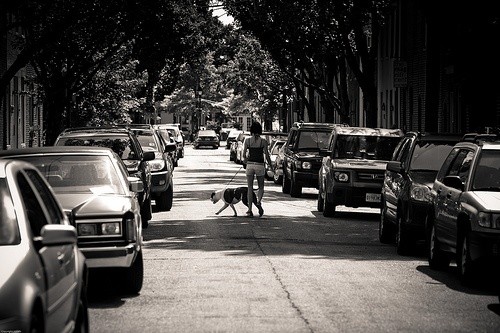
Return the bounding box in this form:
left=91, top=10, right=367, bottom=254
left=247, top=210, right=253, bottom=217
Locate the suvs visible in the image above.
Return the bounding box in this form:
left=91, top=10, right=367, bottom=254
left=282, top=120, right=351, bottom=198
left=318, top=127, right=408, bottom=218
left=54, top=126, right=155, bottom=229
left=378, top=132, right=474, bottom=257
left=423, top=133, right=499, bottom=291
left=109, top=123, right=177, bottom=212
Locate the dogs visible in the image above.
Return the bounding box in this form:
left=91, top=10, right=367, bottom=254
left=209, top=187, right=260, bottom=217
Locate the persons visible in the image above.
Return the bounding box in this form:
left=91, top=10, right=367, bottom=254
left=243, top=122, right=276, bottom=216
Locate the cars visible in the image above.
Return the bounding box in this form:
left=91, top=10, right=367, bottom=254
left=0, top=145, right=145, bottom=296
left=194, top=129, right=220, bottom=149
left=0, top=158, right=89, bottom=333
left=219, top=122, right=289, bottom=185
left=140, top=123, right=197, bottom=167
